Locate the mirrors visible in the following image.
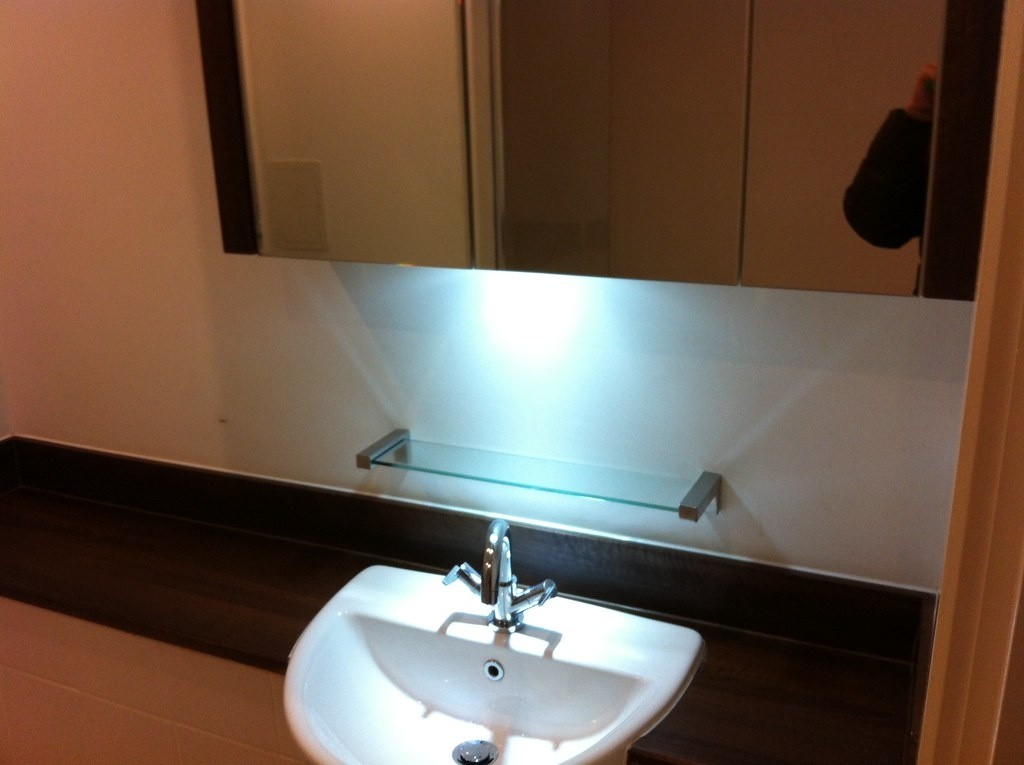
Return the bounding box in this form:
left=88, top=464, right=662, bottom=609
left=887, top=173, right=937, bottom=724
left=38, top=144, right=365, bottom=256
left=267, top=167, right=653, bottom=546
left=192, top=0, right=1006, bottom=302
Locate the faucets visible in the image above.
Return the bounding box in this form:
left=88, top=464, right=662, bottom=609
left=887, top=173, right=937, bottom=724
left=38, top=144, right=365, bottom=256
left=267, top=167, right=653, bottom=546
left=480, top=516, right=519, bottom=630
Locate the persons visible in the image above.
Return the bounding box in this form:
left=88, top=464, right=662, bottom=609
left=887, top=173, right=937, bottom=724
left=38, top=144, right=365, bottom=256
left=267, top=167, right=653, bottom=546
left=842, top=60, right=935, bottom=296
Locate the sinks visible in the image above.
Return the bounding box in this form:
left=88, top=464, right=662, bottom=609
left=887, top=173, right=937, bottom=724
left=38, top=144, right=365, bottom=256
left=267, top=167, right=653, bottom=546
left=282, top=563, right=707, bottom=765
